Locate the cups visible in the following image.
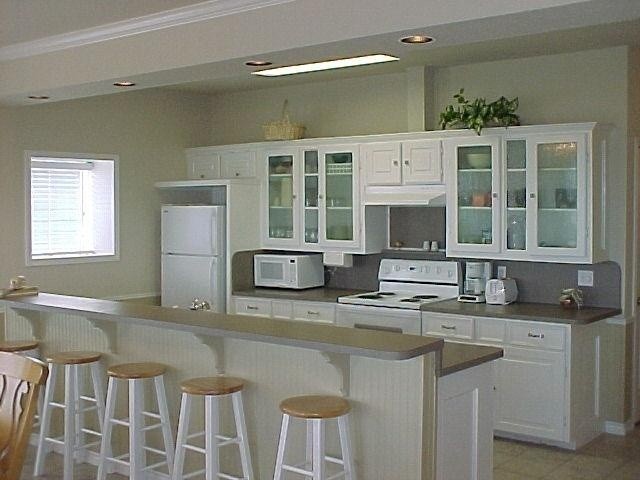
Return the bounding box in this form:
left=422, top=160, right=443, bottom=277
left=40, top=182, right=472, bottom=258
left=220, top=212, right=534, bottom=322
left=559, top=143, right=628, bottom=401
left=269, top=226, right=317, bottom=242
left=556, top=189, right=567, bottom=208
left=422, top=240, right=430, bottom=250
left=460, top=191, right=493, bottom=206
left=432, top=240, right=438, bottom=251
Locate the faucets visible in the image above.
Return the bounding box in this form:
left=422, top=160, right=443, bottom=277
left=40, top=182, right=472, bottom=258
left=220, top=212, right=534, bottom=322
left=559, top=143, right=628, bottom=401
left=188, top=297, right=211, bottom=312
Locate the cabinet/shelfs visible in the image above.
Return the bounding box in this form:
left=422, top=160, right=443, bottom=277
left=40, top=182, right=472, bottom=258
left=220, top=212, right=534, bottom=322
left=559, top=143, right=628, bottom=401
left=422, top=311, right=620, bottom=452
left=185, top=142, right=257, bottom=180
left=360, top=130, right=446, bottom=191
left=263, top=134, right=390, bottom=255
left=446, top=122, right=611, bottom=263
left=232, top=294, right=336, bottom=326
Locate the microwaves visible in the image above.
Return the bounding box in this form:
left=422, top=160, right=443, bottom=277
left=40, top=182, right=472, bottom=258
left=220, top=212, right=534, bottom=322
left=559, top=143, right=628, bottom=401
left=253, top=253, right=325, bottom=292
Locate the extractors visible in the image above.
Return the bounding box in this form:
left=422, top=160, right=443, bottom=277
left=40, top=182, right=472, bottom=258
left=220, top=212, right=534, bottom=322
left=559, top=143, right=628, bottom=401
left=362, top=186, right=446, bottom=209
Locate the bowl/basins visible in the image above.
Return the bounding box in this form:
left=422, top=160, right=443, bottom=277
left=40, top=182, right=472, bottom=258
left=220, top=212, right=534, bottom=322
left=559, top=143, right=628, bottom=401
left=465, top=152, right=492, bottom=168
left=333, top=155, right=350, bottom=163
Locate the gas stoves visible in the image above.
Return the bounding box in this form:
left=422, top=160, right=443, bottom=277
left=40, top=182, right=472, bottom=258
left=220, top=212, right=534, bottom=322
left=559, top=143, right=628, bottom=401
left=337, top=281, right=459, bottom=309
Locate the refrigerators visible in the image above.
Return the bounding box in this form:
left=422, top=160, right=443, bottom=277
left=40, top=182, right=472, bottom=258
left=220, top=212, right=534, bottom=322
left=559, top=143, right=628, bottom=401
left=161, top=202, right=226, bottom=311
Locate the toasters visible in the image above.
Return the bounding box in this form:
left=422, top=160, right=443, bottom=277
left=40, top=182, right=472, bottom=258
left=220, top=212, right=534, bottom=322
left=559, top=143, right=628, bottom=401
left=485, top=277, right=518, bottom=305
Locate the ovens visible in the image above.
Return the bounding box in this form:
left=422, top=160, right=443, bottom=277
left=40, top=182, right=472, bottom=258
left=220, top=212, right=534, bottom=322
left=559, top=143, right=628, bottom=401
left=336, top=304, right=421, bottom=335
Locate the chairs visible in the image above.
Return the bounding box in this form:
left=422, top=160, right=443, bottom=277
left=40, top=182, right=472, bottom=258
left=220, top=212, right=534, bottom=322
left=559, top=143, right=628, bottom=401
left=0, top=348, right=50, bottom=480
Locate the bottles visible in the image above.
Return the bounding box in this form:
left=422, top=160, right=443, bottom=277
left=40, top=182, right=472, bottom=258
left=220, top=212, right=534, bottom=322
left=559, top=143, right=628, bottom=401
left=482, top=229, right=491, bottom=243
left=509, top=215, right=525, bottom=250
left=280, top=178, right=293, bottom=207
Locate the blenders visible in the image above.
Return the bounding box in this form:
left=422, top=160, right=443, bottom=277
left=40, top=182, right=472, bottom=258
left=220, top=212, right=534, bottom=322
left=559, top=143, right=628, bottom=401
left=458, top=261, right=486, bottom=303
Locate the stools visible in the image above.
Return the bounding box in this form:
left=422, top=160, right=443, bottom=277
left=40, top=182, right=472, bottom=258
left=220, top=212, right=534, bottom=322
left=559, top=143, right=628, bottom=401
left=168, top=373, right=258, bottom=480
left=272, top=394, right=356, bottom=480
left=96, top=361, right=175, bottom=479
left=0, top=338, right=44, bottom=443
left=33, top=350, right=115, bottom=480
